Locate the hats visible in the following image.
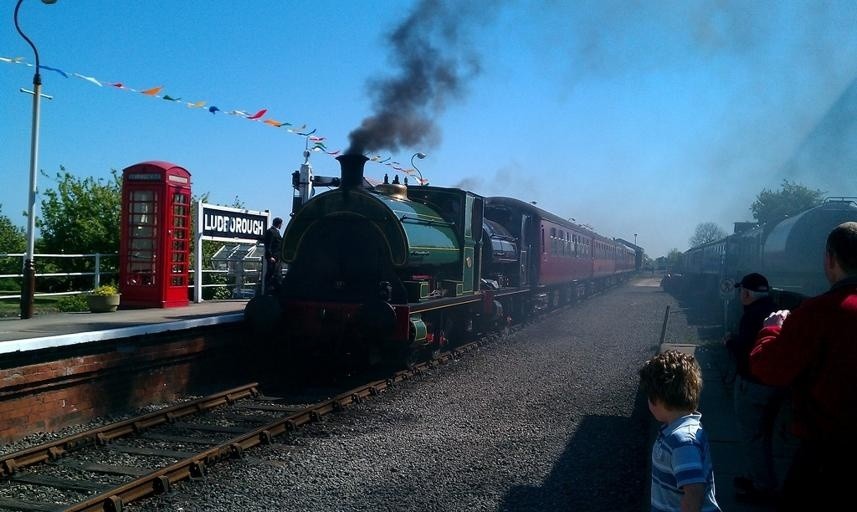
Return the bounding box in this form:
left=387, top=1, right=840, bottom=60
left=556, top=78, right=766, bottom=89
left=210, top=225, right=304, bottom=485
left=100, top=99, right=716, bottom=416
left=735, top=273, right=769, bottom=294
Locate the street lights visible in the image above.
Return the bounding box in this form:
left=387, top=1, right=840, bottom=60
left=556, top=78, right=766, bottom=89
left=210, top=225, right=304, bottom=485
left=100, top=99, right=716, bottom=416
left=632, top=233, right=638, bottom=245
left=407, top=150, right=429, bottom=186
left=567, top=217, right=576, bottom=222
left=12, top=0, right=62, bottom=319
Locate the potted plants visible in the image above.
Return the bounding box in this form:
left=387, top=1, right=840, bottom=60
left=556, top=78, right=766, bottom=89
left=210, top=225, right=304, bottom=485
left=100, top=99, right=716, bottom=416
left=86, top=284, right=123, bottom=313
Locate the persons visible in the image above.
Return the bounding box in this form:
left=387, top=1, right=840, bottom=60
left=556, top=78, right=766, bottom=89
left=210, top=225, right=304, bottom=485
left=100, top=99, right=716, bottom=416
left=722, top=272, right=790, bottom=504
left=264, top=217, right=283, bottom=291
left=640, top=348, right=723, bottom=512
left=749, top=218, right=855, bottom=512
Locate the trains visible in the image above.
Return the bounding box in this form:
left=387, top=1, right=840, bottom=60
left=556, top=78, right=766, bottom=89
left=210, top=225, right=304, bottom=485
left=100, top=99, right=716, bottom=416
left=239, top=142, right=644, bottom=387
left=680, top=191, right=857, bottom=320
